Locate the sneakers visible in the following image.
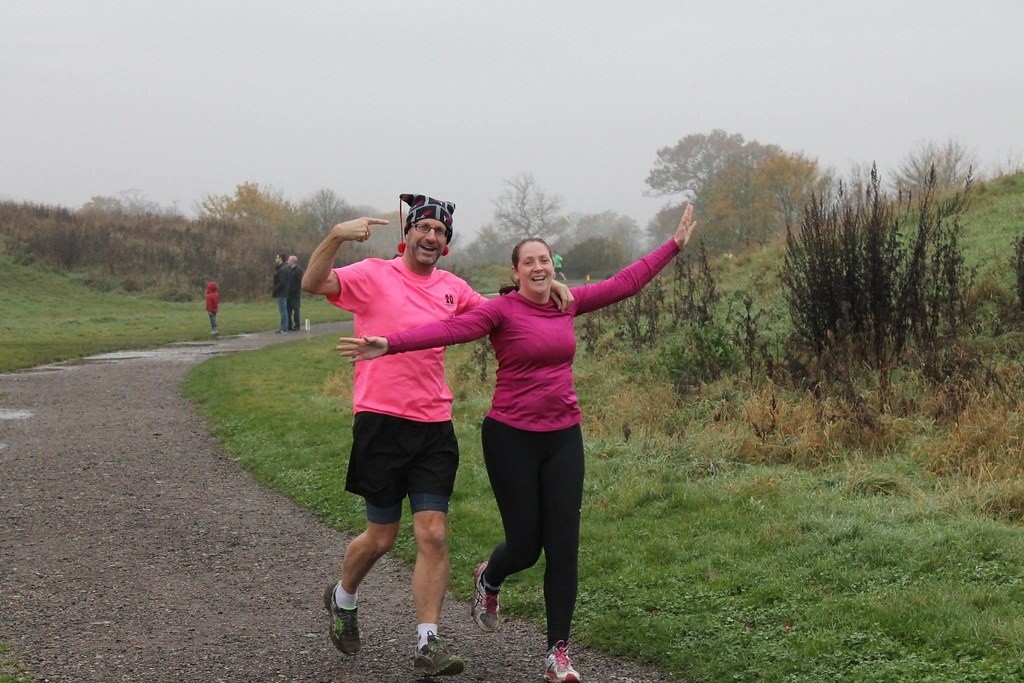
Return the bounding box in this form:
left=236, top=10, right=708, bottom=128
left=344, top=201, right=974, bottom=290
left=323, top=578, right=362, bottom=656
left=472, top=561, right=501, bottom=632
left=542, top=639, right=581, bottom=683
left=413, top=630, right=464, bottom=677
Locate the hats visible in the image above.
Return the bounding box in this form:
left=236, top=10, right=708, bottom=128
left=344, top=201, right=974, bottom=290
left=397, top=193, right=455, bottom=256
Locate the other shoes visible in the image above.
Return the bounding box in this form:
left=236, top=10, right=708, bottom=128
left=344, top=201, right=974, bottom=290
left=275, top=330, right=289, bottom=334
left=208, top=329, right=220, bottom=335
left=292, top=326, right=300, bottom=331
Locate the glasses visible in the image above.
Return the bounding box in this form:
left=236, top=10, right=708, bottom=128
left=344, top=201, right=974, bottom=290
left=410, top=223, right=449, bottom=237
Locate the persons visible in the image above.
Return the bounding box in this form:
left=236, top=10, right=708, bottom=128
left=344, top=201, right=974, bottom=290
left=206, top=282, right=219, bottom=334
left=337, top=203, right=697, bottom=676
left=287, top=255, right=303, bottom=331
left=552, top=250, right=566, bottom=281
left=302, top=192, right=574, bottom=675
left=273, top=253, right=290, bottom=334
